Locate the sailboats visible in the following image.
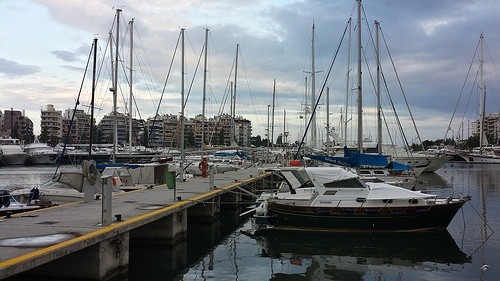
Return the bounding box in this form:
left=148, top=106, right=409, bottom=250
left=0, top=9, right=262, bottom=191
left=295, top=0, right=453, bottom=192
left=437, top=32, right=500, bottom=163
left=9, top=37, right=126, bottom=206
left=240, top=0, right=472, bottom=232
